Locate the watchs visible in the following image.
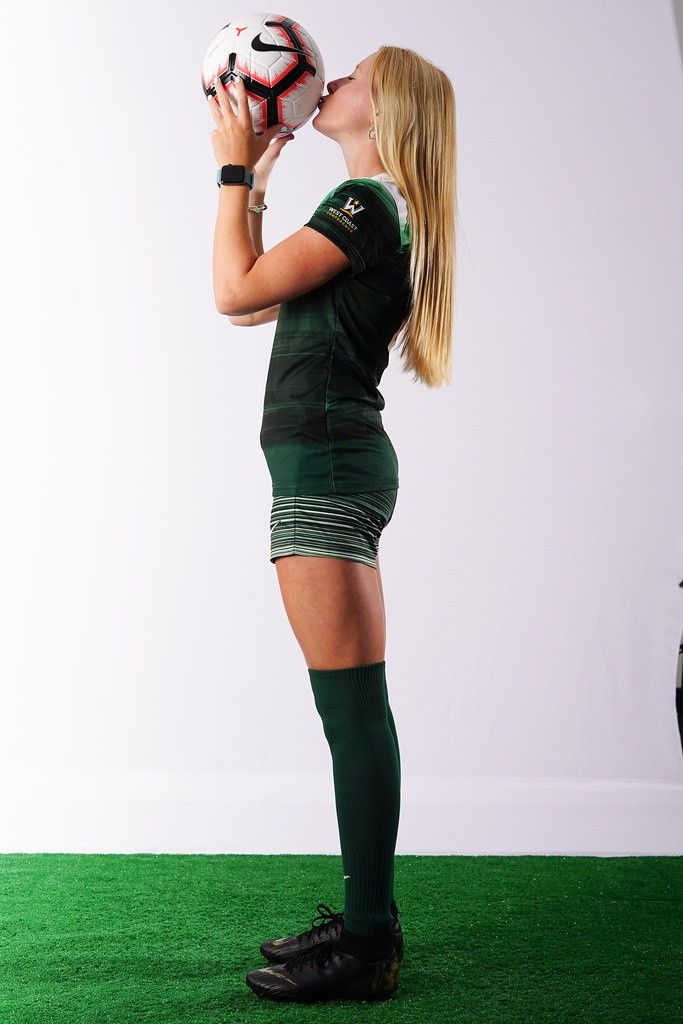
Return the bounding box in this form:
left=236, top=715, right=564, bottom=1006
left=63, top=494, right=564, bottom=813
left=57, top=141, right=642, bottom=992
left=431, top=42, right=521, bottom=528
left=216, top=164, right=255, bottom=190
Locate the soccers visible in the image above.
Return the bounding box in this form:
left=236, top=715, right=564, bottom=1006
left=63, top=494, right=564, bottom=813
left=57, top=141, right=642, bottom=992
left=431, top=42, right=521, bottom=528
left=203, top=11, right=325, bottom=136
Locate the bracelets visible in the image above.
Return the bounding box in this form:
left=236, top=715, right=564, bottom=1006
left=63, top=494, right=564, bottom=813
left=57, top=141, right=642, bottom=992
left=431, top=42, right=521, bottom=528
left=248, top=204, right=267, bottom=213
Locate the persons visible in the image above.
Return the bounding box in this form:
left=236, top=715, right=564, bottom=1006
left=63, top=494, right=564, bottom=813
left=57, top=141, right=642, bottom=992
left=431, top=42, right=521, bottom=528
left=208, top=44, right=458, bottom=1001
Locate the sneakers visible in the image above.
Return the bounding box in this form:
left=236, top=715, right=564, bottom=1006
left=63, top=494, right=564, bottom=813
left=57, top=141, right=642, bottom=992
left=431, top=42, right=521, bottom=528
left=243, top=915, right=403, bottom=1003
